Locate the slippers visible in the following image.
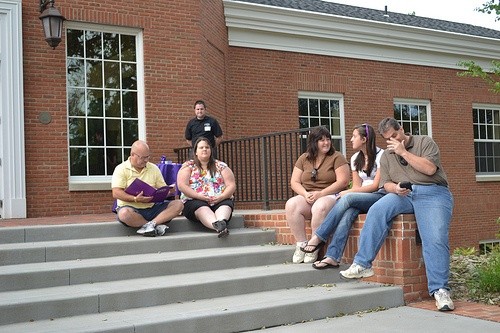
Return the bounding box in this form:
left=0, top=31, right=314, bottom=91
left=299, top=241, right=324, bottom=253
left=312, top=259, right=339, bottom=268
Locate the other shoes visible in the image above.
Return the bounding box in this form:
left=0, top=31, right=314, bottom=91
left=212, top=219, right=230, bottom=238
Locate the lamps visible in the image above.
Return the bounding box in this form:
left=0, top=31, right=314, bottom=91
left=39, top=0, right=68, bottom=51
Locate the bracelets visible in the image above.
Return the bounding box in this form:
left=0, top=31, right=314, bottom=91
left=134, top=196, right=137, bottom=203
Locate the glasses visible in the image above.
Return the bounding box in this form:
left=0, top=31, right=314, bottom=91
left=311, top=167, right=317, bottom=182
left=134, top=153, right=150, bottom=160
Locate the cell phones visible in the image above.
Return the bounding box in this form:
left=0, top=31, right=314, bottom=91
left=400, top=181, right=412, bottom=191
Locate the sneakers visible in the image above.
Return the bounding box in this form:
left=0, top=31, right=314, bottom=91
left=291, top=241, right=320, bottom=264
left=136, top=224, right=169, bottom=236
left=434, top=289, right=455, bottom=311
left=340, top=263, right=374, bottom=279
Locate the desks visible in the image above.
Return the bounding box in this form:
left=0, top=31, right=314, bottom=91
left=156, top=163, right=182, bottom=200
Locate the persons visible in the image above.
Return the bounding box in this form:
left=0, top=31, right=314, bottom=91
left=111, top=140, right=184, bottom=236
left=285, top=126, right=350, bottom=262
left=299, top=123, right=385, bottom=269
left=183, top=100, right=224, bottom=160
left=176, top=136, right=236, bottom=239
left=340, top=116, right=458, bottom=312
